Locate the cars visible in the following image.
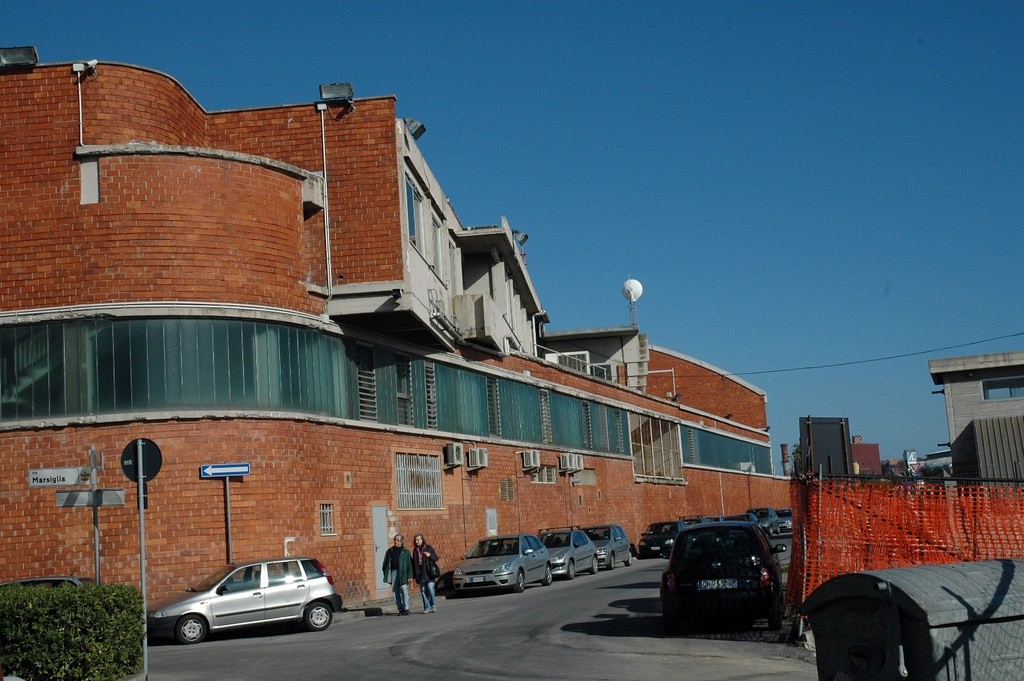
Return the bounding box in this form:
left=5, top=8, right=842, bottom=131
left=576, top=524, right=633, bottom=570
left=676, top=506, right=793, bottom=537
left=452, top=533, right=553, bottom=595
left=536, top=525, right=599, bottom=580
left=659, top=521, right=788, bottom=633
left=638, top=521, right=687, bottom=559
left=0, top=575, right=97, bottom=591
left=146, top=555, right=344, bottom=645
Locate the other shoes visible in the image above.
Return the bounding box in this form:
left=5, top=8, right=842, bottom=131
left=405, top=610, right=410, bottom=614
left=398, top=610, right=403, bottom=616
left=432, top=607, right=437, bottom=613
left=422, top=609, right=431, bottom=614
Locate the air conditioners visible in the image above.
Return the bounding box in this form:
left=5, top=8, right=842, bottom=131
left=469, top=448, right=488, bottom=467
left=524, top=450, right=540, bottom=468
left=560, top=453, right=584, bottom=470
left=447, top=442, right=464, bottom=466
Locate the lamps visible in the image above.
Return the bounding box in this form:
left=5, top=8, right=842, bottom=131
left=404, top=116, right=427, bottom=140
left=512, top=229, right=529, bottom=246
left=672, top=393, right=681, bottom=401
left=319, top=82, right=356, bottom=120
left=0, top=46, right=38, bottom=68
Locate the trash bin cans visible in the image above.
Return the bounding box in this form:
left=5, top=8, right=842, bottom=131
left=798, top=562, right=1024, bottom=681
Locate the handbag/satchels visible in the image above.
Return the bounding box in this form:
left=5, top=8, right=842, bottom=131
left=426, top=558, right=440, bottom=578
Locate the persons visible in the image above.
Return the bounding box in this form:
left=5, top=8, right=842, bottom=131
left=412, top=534, right=440, bottom=614
left=381, top=533, right=414, bottom=616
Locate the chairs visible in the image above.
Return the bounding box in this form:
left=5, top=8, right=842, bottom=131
left=512, top=542, right=519, bottom=551
left=255, top=571, right=261, bottom=579
left=490, top=545, right=500, bottom=553
left=227, top=577, right=234, bottom=584
left=554, top=537, right=562, bottom=543
left=566, top=535, right=570, bottom=545
left=603, top=530, right=609, bottom=536
left=591, top=534, right=600, bottom=539
